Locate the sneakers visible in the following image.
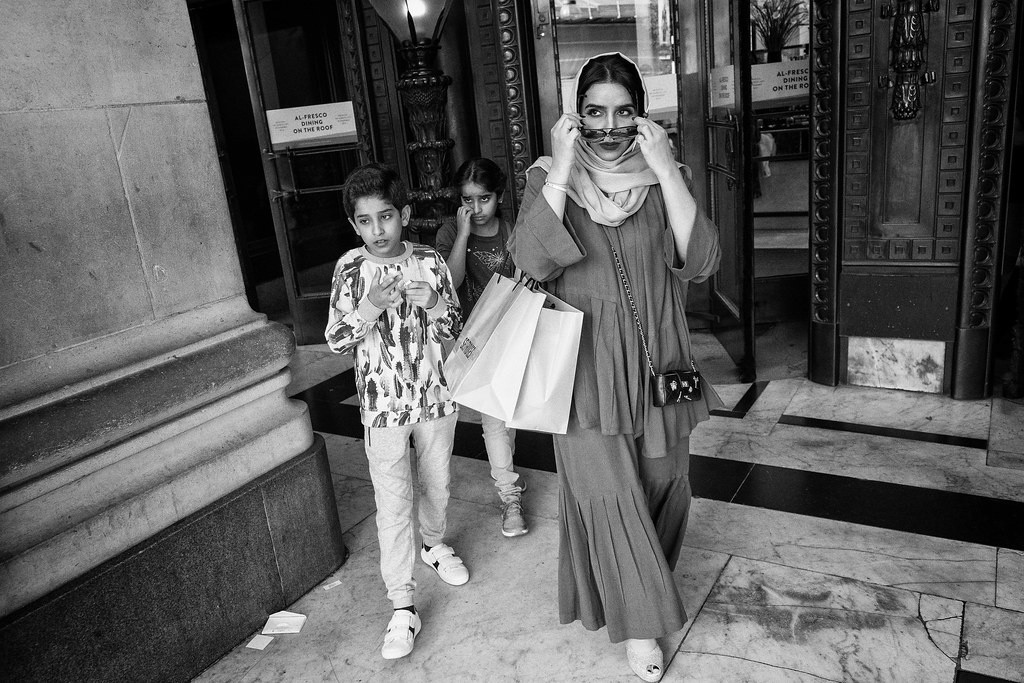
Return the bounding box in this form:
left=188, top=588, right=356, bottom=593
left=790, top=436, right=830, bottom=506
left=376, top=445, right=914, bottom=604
left=498, top=496, right=528, bottom=537
left=421, top=542, right=469, bottom=586
left=381, top=609, right=421, bottom=659
left=491, top=467, right=526, bottom=491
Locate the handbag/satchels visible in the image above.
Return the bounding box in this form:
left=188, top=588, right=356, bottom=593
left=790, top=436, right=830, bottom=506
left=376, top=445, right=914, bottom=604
left=651, top=370, right=702, bottom=408
left=443, top=251, right=585, bottom=434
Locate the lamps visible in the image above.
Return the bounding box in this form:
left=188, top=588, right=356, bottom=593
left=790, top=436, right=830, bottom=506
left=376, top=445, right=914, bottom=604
left=370, top=0, right=456, bottom=86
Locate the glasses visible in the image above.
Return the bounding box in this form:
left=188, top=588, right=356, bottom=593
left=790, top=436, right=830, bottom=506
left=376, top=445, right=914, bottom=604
left=578, top=88, right=639, bottom=139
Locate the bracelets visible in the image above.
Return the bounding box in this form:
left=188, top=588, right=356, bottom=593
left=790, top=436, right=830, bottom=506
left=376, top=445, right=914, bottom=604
left=544, top=182, right=568, bottom=193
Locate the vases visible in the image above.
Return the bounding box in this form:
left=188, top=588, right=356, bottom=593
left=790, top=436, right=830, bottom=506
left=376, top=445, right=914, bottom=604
left=767, top=48, right=782, bottom=64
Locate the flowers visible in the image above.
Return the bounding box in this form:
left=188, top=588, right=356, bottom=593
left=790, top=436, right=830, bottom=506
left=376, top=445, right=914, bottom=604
left=749, top=0, right=810, bottom=48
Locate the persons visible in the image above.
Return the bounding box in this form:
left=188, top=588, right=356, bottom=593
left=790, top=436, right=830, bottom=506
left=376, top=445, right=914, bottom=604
left=506, top=51, right=724, bottom=681
left=324, top=163, right=471, bottom=660
left=436, top=159, right=547, bottom=537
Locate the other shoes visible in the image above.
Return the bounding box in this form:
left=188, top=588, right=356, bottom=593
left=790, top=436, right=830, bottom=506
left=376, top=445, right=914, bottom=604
left=624, top=642, right=663, bottom=682
